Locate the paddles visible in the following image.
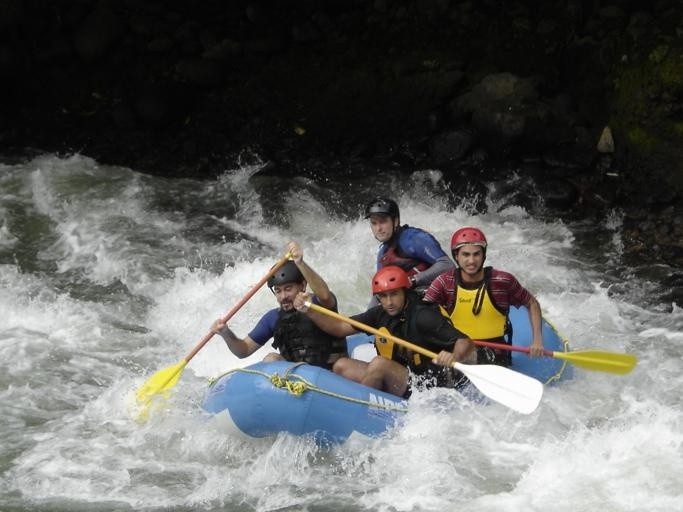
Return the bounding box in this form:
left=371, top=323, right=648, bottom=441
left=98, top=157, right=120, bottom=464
left=466, top=339, right=639, bottom=375
left=295, top=291, right=545, bottom=415
left=131, top=243, right=301, bottom=425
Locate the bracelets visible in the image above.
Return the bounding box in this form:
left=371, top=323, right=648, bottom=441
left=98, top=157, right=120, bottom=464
left=409, top=273, right=417, bottom=287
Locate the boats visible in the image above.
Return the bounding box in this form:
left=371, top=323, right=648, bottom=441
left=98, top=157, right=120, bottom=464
left=203, top=305, right=575, bottom=451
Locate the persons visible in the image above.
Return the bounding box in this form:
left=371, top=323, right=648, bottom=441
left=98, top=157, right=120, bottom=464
left=292, top=266, right=477, bottom=400
left=419, top=226, right=546, bottom=370
left=209, top=240, right=348, bottom=374
left=364, top=197, right=456, bottom=312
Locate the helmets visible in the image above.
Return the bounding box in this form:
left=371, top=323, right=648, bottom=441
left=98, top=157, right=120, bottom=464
left=449, top=226, right=487, bottom=251
left=266, top=260, right=305, bottom=288
left=364, top=198, right=400, bottom=220
left=370, top=266, right=411, bottom=295
